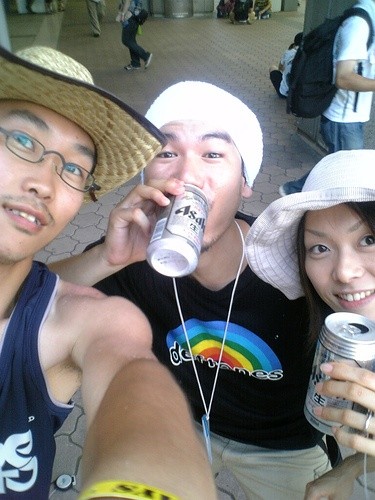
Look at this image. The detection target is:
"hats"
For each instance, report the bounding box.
[244,149,375,301]
[144,81,264,187]
[1,45,168,206]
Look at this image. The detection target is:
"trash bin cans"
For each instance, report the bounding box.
[165,0,193,17]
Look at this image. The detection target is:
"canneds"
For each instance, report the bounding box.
[146,184,208,278]
[304,312,375,437]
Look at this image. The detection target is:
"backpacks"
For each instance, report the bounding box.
[286,7,374,118]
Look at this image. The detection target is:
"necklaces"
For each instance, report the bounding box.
[173,218,245,463]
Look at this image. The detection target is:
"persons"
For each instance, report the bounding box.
[115,1,152,71]
[243,149,375,500]
[86,1,105,37]
[44,81,343,499]
[0,45,217,500]
[24,0,52,15]
[279,0,375,196]
[270,32,303,99]
[216,0,272,25]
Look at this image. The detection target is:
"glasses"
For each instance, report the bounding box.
[0,126,101,192]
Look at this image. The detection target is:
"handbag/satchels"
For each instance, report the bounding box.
[137,10,148,25]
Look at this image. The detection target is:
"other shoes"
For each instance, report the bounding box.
[144,53,153,68]
[124,63,141,70]
[262,15,270,19]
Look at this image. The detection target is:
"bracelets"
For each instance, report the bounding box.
[76,480,182,500]
